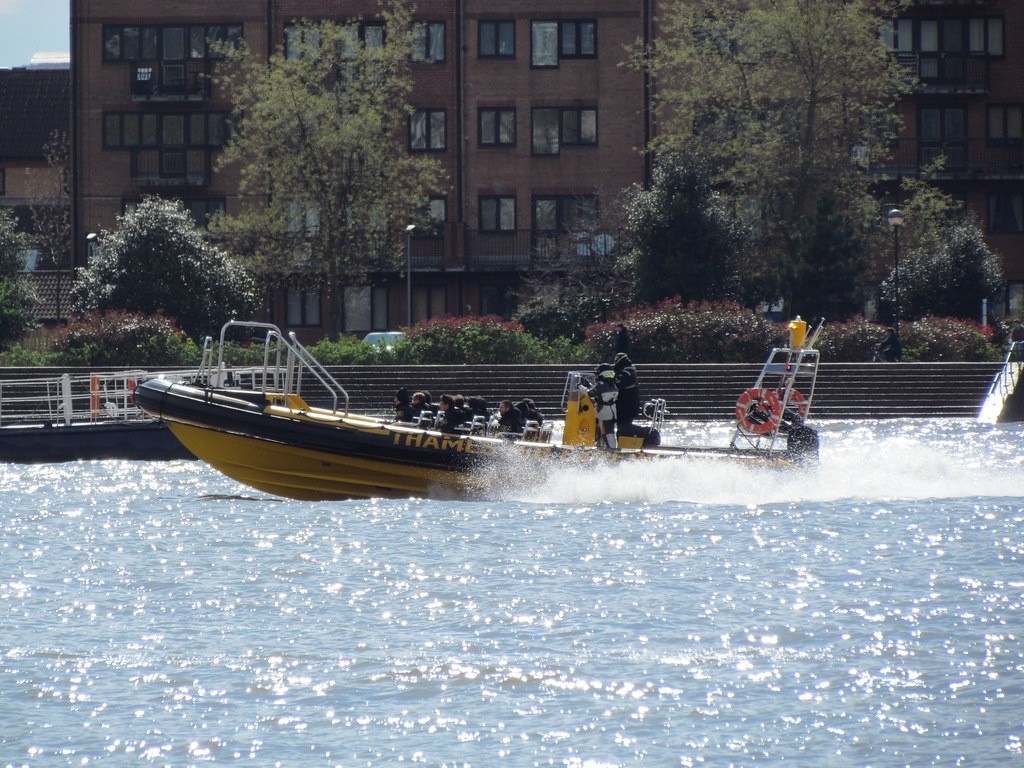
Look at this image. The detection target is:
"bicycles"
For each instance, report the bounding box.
[867,343,918,362]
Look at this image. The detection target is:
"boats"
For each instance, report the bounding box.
[130,313,828,502]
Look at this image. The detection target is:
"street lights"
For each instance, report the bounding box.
[886,209,907,341]
[405,224,416,331]
[86,232,100,348]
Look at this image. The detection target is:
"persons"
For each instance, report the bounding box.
[1011,318,1024,361]
[879,326,902,362]
[422,389,440,426]
[516,398,542,436]
[614,324,632,354]
[453,394,473,421]
[395,387,420,424]
[498,401,525,440]
[410,392,432,427]
[582,370,619,451]
[469,397,490,422]
[439,394,465,434]
[613,351,662,446]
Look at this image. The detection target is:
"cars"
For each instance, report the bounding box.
[358,330,412,357]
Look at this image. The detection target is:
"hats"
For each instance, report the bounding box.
[594,363,610,374]
[396,388,408,403]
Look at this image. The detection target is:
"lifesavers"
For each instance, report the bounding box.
[735,387,781,435]
[771,386,807,434]
